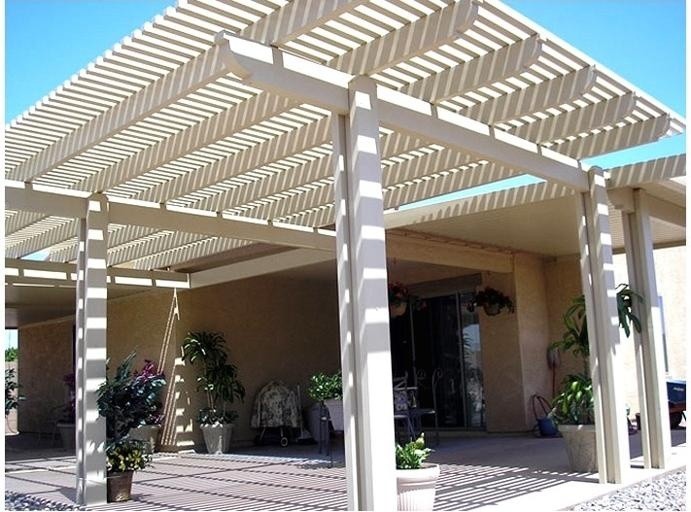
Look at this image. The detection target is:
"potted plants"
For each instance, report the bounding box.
[549,374,597,473]
[106,438,152,503]
[394,432,439,512]
[309,368,344,433]
[181,328,246,454]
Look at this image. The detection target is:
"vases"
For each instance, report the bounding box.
[127,422,161,456]
[56,421,75,451]
[390,301,407,315]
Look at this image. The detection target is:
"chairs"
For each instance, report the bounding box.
[393,389,440,449]
[316,402,330,456]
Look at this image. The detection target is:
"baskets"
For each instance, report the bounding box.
[390,302,407,318]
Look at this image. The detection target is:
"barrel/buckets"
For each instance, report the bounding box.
[536,417,556,436]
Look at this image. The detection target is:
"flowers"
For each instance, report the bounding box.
[55,374,78,425]
[388,280,426,310]
[464,287,514,315]
[130,357,165,425]
